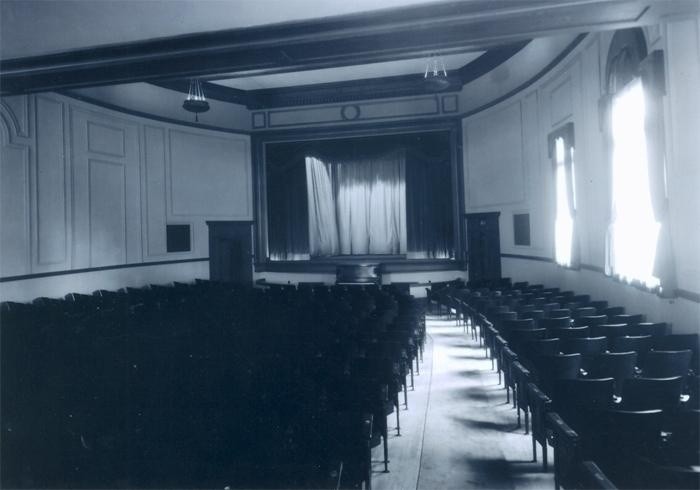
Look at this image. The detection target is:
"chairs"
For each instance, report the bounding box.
[2,277,427,490]
[421,270,700,490]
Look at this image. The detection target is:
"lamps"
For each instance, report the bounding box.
[422,58,450,93]
[184,78,210,123]
